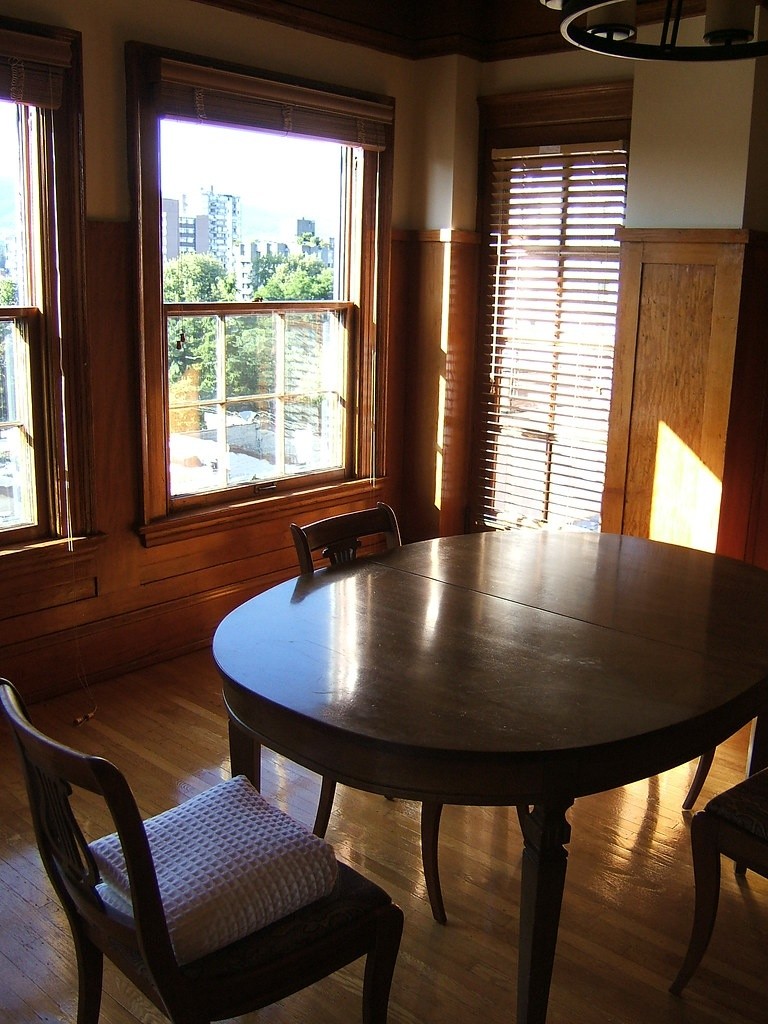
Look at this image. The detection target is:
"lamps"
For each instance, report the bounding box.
[540,0,768,61]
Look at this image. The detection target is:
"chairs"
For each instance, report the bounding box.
[667,767,768,996]
[293,502,448,926]
[0,676,404,1024]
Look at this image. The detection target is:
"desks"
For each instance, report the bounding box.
[210,529,768,1024]
